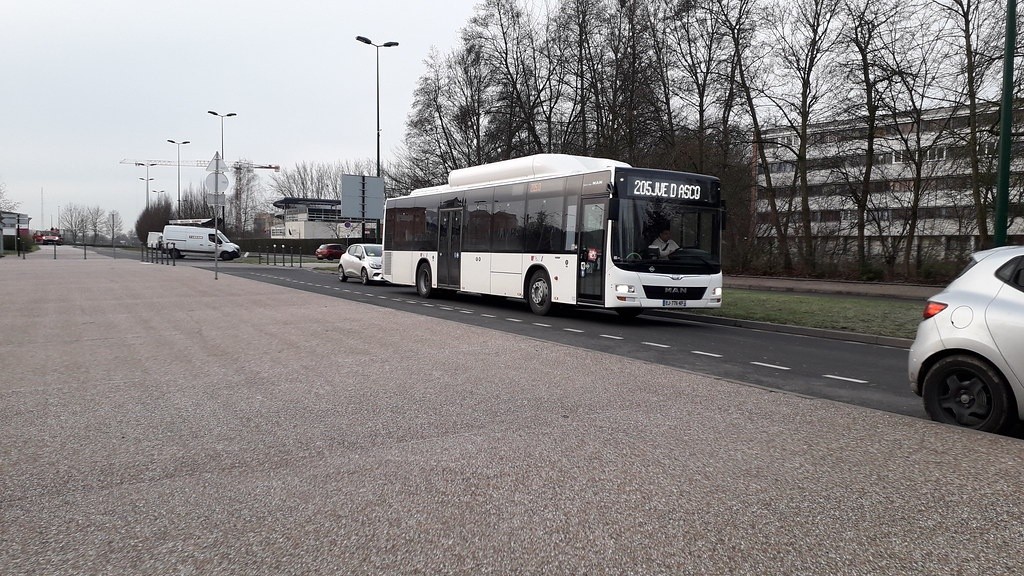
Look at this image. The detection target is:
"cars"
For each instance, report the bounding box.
[33,231,61,245]
[907,246,1024,435]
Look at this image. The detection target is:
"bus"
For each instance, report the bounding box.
[381,153,728,322]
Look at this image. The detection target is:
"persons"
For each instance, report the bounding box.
[651,228,681,260]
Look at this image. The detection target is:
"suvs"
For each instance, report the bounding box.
[337,244,390,286]
[316,244,346,261]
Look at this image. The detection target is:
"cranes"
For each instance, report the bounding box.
[120,156,281,234]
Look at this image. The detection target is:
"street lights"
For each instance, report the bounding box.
[208,111,237,162]
[356,34,399,177]
[167,140,190,221]
[152,190,164,207]
[140,177,154,210]
[138,162,156,212]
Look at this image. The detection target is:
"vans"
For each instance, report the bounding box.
[147,232,163,249]
[161,218,242,261]
